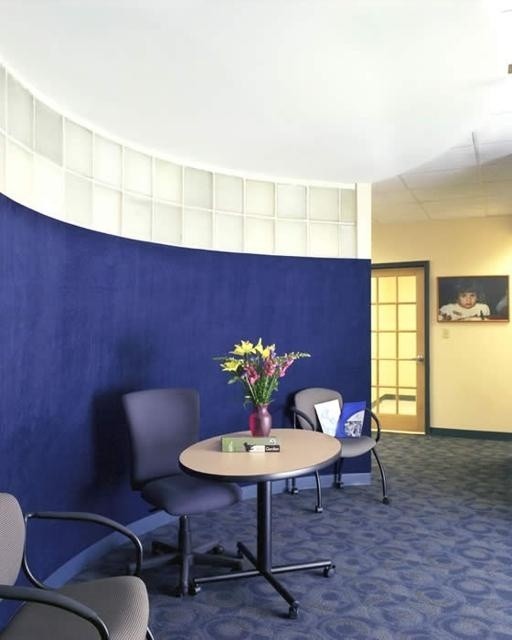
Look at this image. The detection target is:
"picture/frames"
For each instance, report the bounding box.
[436,275,510,323]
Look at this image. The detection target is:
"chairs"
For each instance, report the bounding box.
[122,389,244,597]
[0,492,153,639]
[292,387,390,513]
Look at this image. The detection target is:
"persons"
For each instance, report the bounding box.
[439,285,491,321]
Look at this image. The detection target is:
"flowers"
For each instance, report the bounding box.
[212,337,312,435]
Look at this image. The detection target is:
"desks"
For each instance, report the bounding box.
[179,427,342,620]
